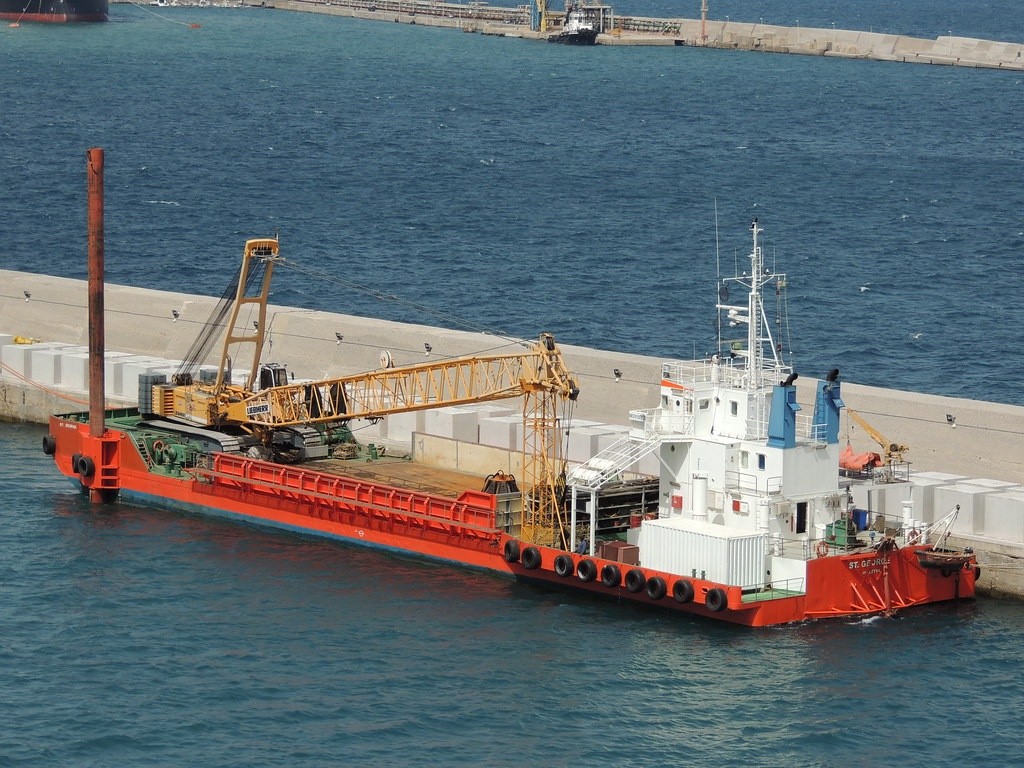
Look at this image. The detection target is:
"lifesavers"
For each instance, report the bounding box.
[672,579,695,603]
[153,440,164,453]
[601,564,621,588]
[77,456,93,476]
[410,20,416,25]
[941,567,953,577]
[9,20,20,31]
[645,577,667,600]
[395,18,398,22]
[624,569,645,594]
[553,554,574,578]
[42,435,56,454]
[505,540,520,563]
[812,540,832,559]
[71,454,83,474]
[409,12,415,17]
[975,564,982,582]
[521,547,541,570]
[366,5,376,13]
[186,20,202,31]
[704,587,728,613]
[905,532,921,544]
[576,559,597,582]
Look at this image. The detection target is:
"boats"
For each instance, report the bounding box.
[546,0,601,45]
[40,146,981,627]
[0,0,107,22]
